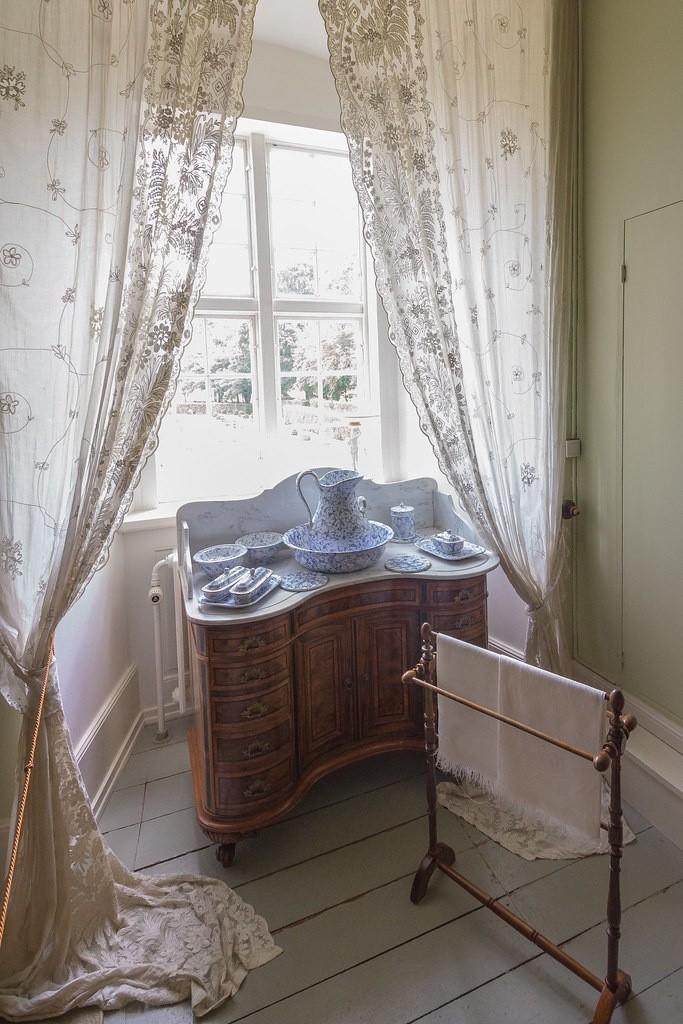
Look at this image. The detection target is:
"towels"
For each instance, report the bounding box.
[429,631,610,848]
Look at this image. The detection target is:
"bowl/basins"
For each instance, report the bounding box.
[283,520,394,573]
[235,532,283,566]
[193,544,248,580]
[432,530,465,555]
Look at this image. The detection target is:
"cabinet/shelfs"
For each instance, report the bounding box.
[174,466,502,869]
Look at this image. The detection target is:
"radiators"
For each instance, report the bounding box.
[148,552,193,746]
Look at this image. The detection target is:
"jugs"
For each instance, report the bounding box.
[296,470,373,550]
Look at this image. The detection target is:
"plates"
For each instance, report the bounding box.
[415,539,486,560]
[198,575,282,608]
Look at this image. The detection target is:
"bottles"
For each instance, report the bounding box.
[391,503,415,540]
